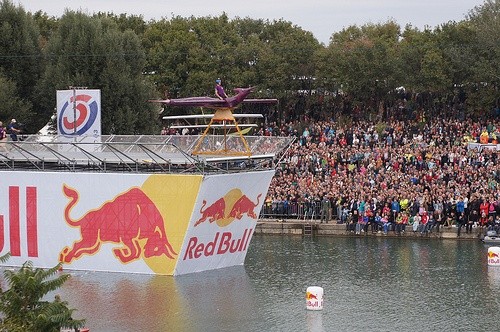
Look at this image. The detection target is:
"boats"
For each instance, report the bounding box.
[0,128,299,277]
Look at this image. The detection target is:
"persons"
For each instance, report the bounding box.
[7,119,21,141]
[215,78,229,100]
[252,118,500,239]
[161,121,251,170]
[0,120,7,141]
